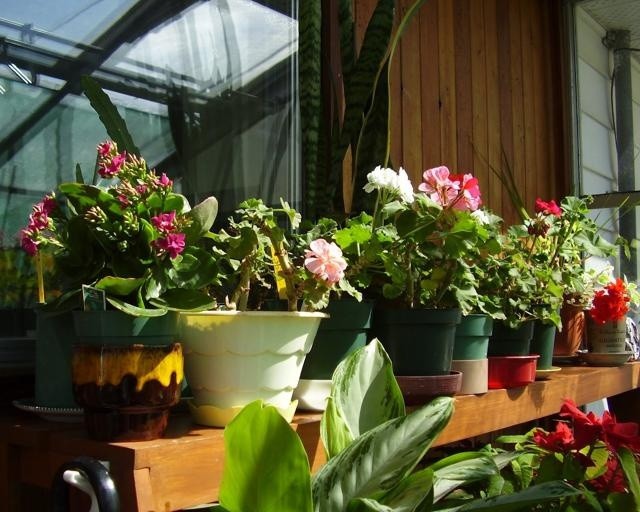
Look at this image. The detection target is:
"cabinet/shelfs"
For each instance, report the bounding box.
[104,357,640,512]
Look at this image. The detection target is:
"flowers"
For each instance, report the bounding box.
[589,277,632,323]
[528,197,561,236]
[21,142,193,269]
[550,404,638,490]
[304,241,348,284]
[362,160,483,219]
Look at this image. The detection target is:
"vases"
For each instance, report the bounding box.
[533,316,559,378]
[458,315,494,396]
[304,286,373,414]
[591,319,634,365]
[59,297,186,440]
[370,303,462,396]
[486,314,541,388]
[553,290,586,366]
[185,283,318,425]
